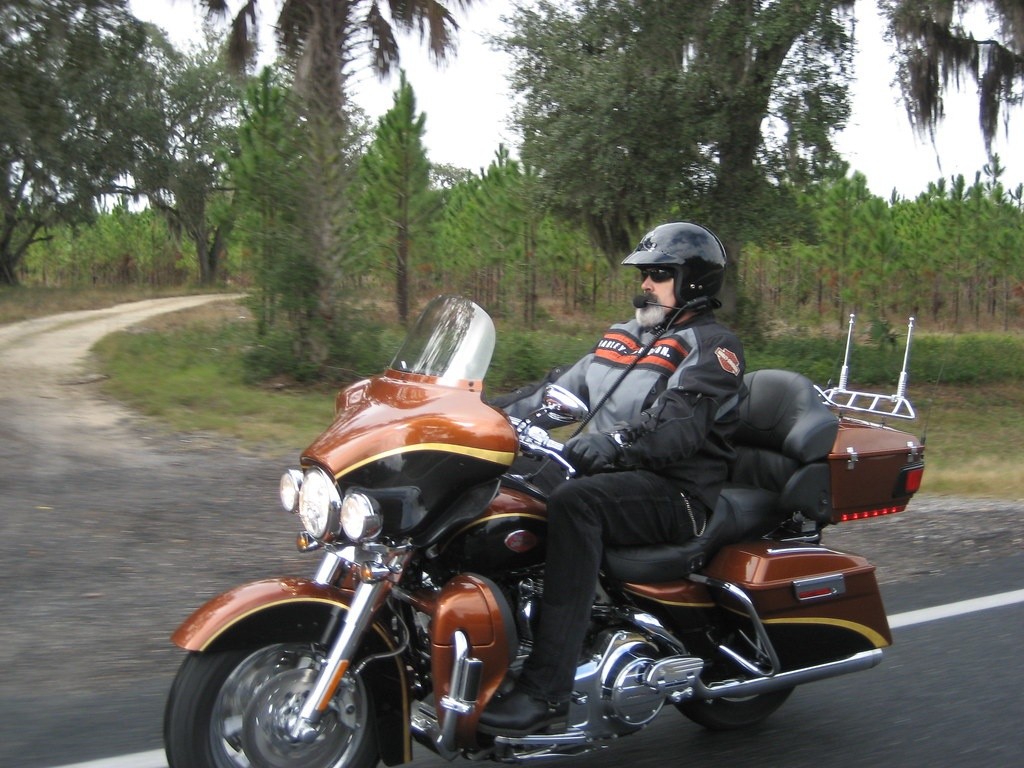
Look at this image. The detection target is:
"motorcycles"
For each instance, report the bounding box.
[163,295,946,768]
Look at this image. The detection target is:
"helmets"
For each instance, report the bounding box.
[621,222,727,311]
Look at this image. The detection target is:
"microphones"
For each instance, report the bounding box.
[633,295,706,312]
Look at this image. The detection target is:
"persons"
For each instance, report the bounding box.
[475,221,750,738]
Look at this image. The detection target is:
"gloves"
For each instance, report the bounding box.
[561,420,637,477]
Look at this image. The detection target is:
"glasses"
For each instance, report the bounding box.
[640,266,675,283]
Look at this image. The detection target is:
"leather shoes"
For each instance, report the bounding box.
[477,689,570,738]
